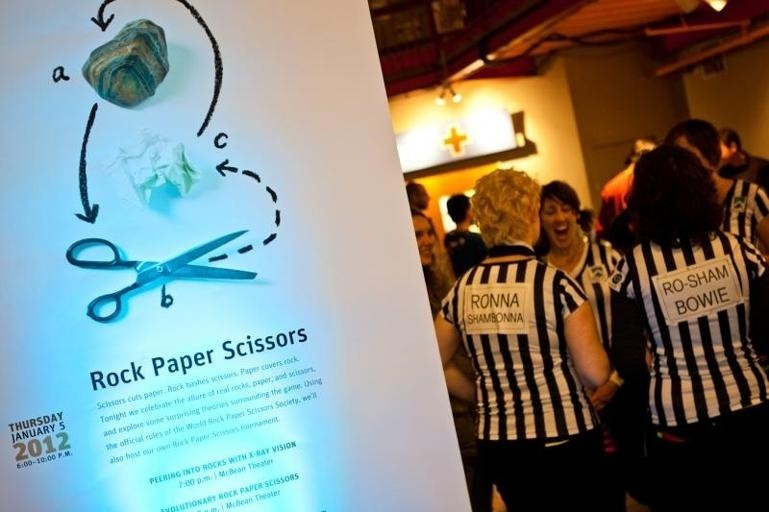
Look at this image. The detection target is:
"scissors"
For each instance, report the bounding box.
[65,230,258,322]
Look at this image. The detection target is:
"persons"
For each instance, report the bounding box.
[405,117,768,511]
